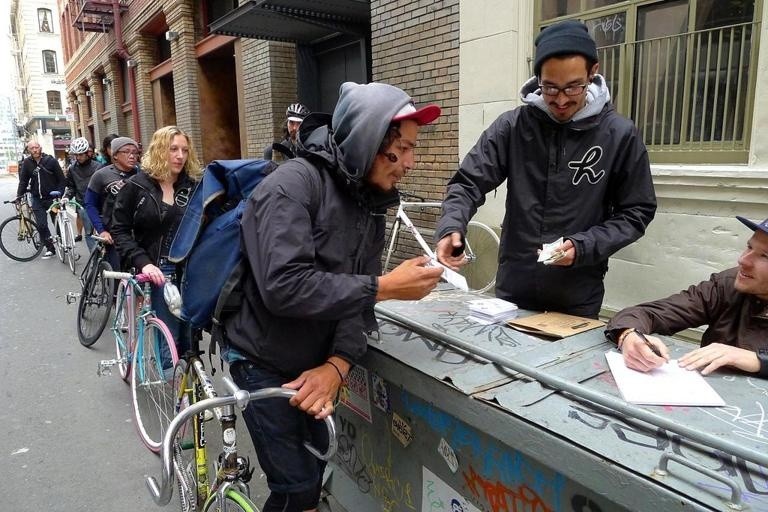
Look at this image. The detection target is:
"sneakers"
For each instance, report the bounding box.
[42,235,82,259]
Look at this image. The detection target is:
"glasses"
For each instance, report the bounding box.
[537,75,593,96]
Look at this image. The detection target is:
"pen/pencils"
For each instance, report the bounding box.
[634,328,668,365]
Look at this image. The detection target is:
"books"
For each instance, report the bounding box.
[604,350,726,407]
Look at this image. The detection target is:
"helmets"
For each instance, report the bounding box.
[286,103,311,119]
[68,136,89,155]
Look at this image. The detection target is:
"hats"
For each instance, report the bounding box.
[534,22,598,77]
[111,137,140,162]
[736,215,768,234]
[393,102,441,126]
[287,116,303,122]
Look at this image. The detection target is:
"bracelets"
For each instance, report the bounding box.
[612,327,641,351]
[323,359,344,383]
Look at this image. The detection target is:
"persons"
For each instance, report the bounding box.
[15,125,218,425]
[605,209,768,380]
[434,17,656,322]
[261,101,313,164]
[210,78,444,511]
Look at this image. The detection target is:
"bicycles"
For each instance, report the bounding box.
[0,188,45,263]
[145,323,338,511]
[102,267,190,453]
[45,191,85,275]
[383,185,502,293]
[65,233,115,348]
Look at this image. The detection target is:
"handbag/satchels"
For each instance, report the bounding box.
[169,159,278,332]
